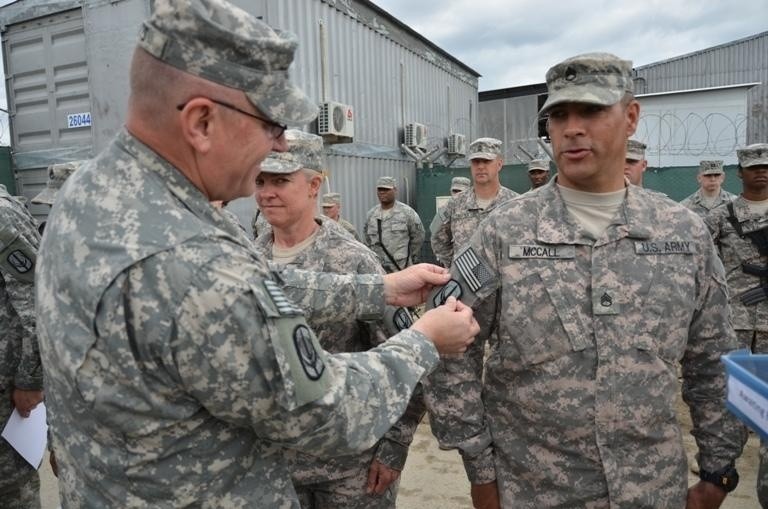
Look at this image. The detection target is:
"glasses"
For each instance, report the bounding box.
[176,100,286,139]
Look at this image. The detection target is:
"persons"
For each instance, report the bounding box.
[622,139,768,354]
[252,131,423,509]
[424,52,750,508]
[34,0,481,508]
[429,137,550,266]
[1,159,90,509]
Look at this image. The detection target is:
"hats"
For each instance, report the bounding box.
[31,160,89,203]
[527,159,549,172]
[624,140,646,161]
[699,161,722,176]
[376,177,397,191]
[450,177,470,191]
[735,143,767,168]
[538,52,632,115]
[259,129,323,173]
[137,0,319,127]
[319,192,340,207]
[467,138,501,161]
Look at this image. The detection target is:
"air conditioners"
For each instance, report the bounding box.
[403,121,428,150]
[317,99,354,137]
[447,133,467,156]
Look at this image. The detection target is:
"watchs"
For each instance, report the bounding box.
[700,468,739,491]
